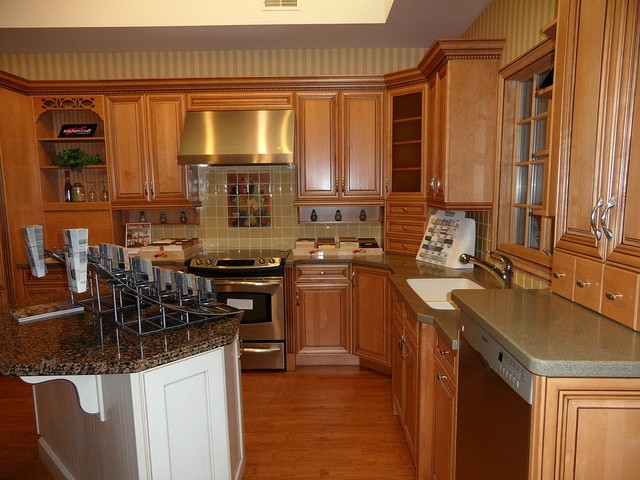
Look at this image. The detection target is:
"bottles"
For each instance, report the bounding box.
[65,170,72,202]
[335,209,343,221]
[310,209,318,222]
[139,211,146,225]
[360,209,366,221]
[159,212,167,224]
[180,210,187,224]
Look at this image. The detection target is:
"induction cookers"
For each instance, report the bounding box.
[189,249,294,274]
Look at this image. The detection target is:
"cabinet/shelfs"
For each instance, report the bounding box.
[18,258,110,301]
[0,69,114,212]
[346,264,392,375]
[284,263,352,368]
[497,37,556,270]
[550,0,640,334]
[294,74,383,206]
[430,326,458,480]
[105,79,189,210]
[388,274,432,480]
[454,309,639,480]
[383,67,429,257]
[417,41,505,210]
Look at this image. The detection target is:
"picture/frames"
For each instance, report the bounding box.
[125,221,152,249]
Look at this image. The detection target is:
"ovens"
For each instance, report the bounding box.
[190,266,284,348]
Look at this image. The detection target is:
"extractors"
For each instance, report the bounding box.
[174,106,299,168]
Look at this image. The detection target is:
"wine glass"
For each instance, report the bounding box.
[100,181,109,200]
[87,182,98,200]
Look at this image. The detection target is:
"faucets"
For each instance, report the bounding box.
[460,251,514,289]
[405,276,485,310]
